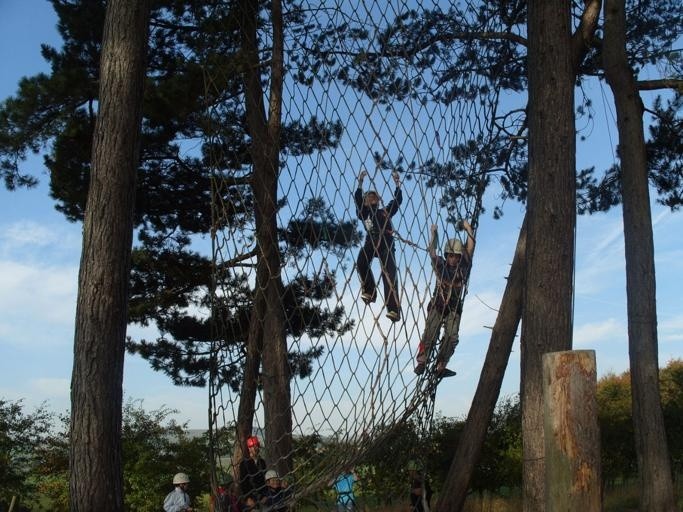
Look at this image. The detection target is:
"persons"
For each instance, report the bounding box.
[237,435,268,510]
[206,468,236,512]
[326,460,362,511]
[413,218,475,380]
[242,495,258,511]
[403,455,433,511]
[351,170,405,324]
[256,466,291,512]
[161,472,194,511]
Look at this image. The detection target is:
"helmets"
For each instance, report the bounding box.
[444,238,463,256]
[172,472,191,485]
[364,190,380,200]
[265,469,280,481]
[246,436,259,447]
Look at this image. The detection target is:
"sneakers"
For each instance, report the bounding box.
[414,363,425,375]
[434,367,456,377]
[360,291,375,302]
[385,309,401,322]
